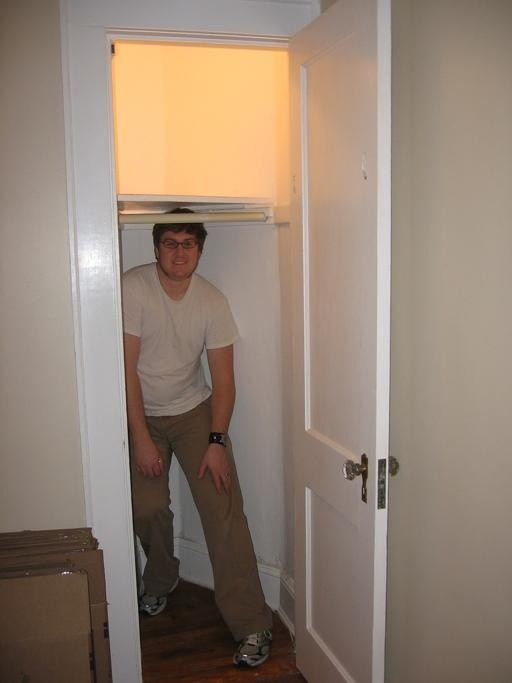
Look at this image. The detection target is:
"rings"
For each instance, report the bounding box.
[225,474,229,481]
[157,458,162,464]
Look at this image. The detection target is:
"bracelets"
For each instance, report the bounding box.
[208,432,228,447]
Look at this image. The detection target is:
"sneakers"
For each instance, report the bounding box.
[139,573,180,617]
[232,629,273,667]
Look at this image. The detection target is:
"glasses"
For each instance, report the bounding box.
[156,237,203,249]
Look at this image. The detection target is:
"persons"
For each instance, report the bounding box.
[123,209,273,667]
